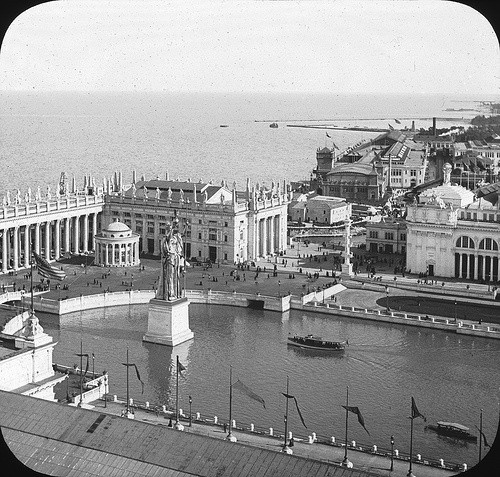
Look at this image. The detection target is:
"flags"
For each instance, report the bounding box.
[31,250,68,281]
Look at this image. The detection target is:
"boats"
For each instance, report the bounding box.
[288,334,345,350]
[426,421,477,439]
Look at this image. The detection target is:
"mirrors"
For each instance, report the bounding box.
[0,0,500,477]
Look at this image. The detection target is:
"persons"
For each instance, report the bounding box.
[155,209,189,300]
